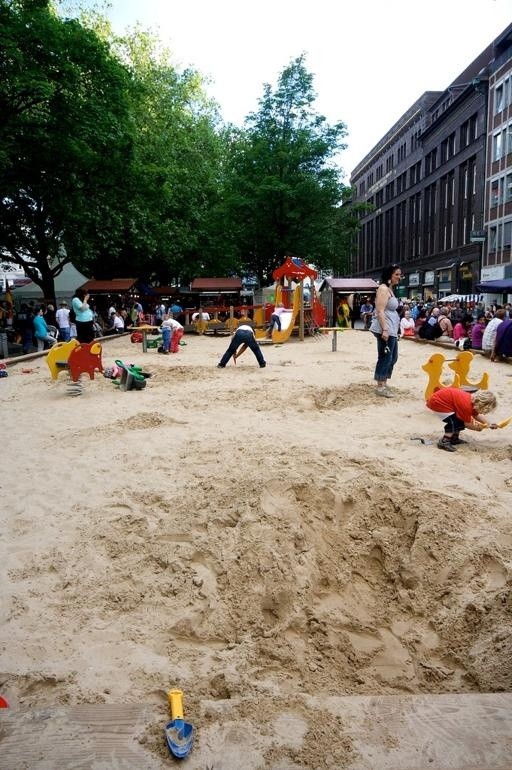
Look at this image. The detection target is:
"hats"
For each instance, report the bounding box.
[59,301,67,305]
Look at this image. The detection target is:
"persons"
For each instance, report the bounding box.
[191,307,210,335]
[0,287,184,360]
[217,324,266,368]
[369,263,400,398]
[267,302,287,337]
[398,295,512,362]
[360,300,373,330]
[426,388,497,452]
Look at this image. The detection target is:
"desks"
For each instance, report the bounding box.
[318,327,352,352]
[126,326,160,353]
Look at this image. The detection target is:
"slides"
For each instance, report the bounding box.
[255,308,299,343]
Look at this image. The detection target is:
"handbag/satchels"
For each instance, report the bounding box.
[418,322,431,338]
[404,327,415,336]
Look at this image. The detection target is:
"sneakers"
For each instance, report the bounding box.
[437,439,456,452]
[376,387,392,398]
[451,438,467,444]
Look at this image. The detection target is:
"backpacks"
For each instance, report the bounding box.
[431,317,447,337]
